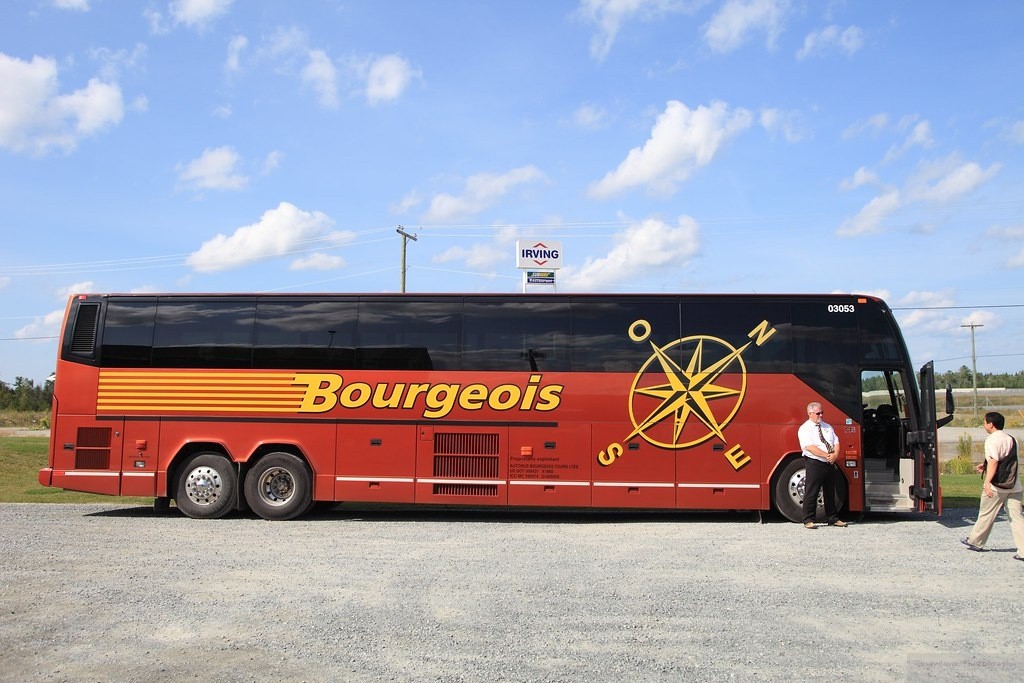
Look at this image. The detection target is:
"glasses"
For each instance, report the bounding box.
[814,411,824,415]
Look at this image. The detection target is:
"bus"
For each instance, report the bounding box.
[39,290,955,519]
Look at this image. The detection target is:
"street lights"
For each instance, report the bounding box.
[959,323,985,416]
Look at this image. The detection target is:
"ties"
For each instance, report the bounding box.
[816,425,839,455]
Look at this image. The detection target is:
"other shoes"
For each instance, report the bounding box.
[833,520,849,526]
[1014,554,1024,561]
[806,521,819,528]
[960,537,983,551]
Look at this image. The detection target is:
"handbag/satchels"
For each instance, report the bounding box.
[980,433,1019,489]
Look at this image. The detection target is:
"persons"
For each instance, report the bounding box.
[960,412,1024,561]
[798,402,848,529]
